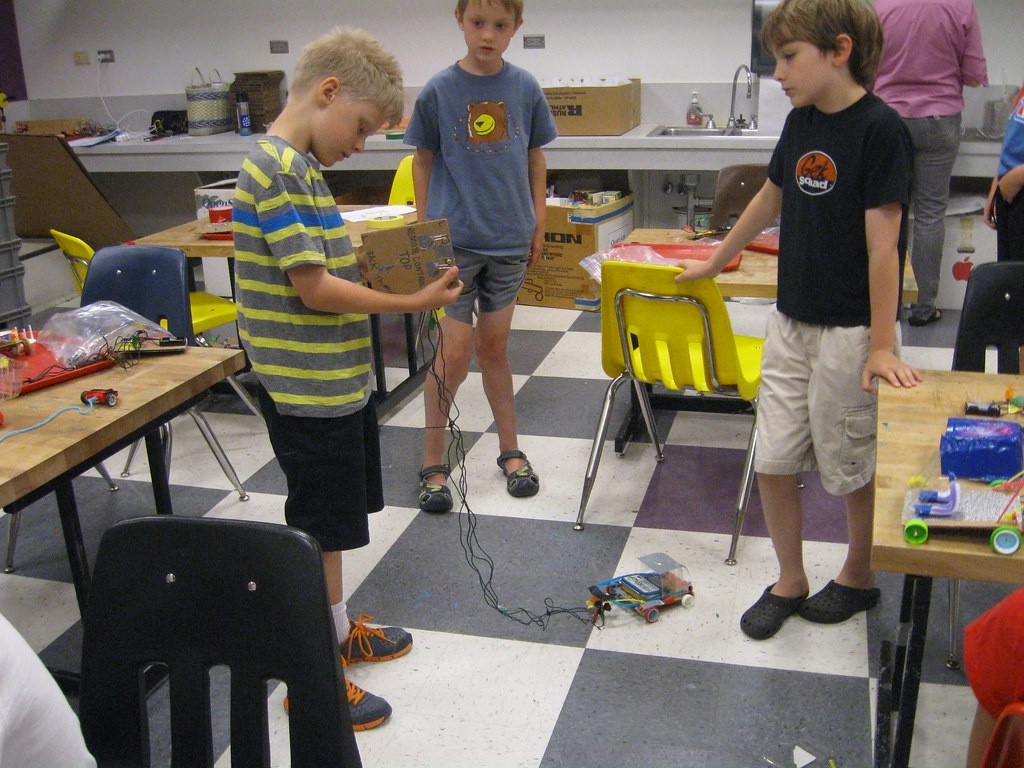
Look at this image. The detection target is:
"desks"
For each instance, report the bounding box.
[123,205,438,414]
[868,369,1024,768]
[0,329,253,629]
[608,228,920,452]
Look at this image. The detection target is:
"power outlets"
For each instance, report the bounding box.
[97,49,115,63]
[523,35,545,49]
[270,41,290,54]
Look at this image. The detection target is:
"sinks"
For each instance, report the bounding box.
[727,128,758,136]
[646,125,727,136]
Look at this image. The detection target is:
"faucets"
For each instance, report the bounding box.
[726,64,753,128]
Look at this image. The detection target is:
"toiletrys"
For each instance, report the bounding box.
[687,91,703,125]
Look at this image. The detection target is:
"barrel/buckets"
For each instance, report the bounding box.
[675,207,712,230]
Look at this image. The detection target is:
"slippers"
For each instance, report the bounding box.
[799,580,881,623]
[741,583,809,641]
[908,308,942,326]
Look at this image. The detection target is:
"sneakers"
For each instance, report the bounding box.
[339,614,413,664]
[284,679,391,731]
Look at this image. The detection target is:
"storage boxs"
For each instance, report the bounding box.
[539,78,640,135]
[515,194,632,311]
[902,196,1000,313]
[0,142,34,332]
[193,178,239,237]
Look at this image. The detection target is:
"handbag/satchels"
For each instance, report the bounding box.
[185,68,235,136]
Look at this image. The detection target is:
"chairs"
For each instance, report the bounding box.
[78,516,363,768]
[574,258,765,566]
[946,261,1023,671]
[385,157,446,356]
[68,244,248,506]
[43,229,271,430]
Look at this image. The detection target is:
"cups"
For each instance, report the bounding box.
[209,207,232,223]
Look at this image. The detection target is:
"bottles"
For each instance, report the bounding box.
[235,92,252,135]
[572,189,584,206]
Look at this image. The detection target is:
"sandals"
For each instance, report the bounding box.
[419,465,453,511]
[496,450,539,497]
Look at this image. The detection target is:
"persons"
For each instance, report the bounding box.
[674,0,923,640]
[0,614,99,768]
[983,86,1024,375]
[403,0,559,515]
[233,28,465,731]
[964,588,1024,768]
[874,1,989,329]
